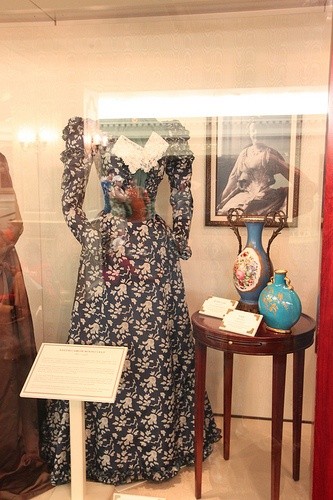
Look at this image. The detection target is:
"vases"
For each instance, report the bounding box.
[226,208,286,311]
[258,269,302,335]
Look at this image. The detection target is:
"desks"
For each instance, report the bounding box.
[189,302,316,500]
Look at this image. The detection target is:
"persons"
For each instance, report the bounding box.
[218,119,288,217]
[0,110,222,500]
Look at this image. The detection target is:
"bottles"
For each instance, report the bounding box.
[233,218,272,304]
[257,270,302,333]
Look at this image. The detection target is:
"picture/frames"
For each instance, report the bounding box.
[204,113,303,230]
